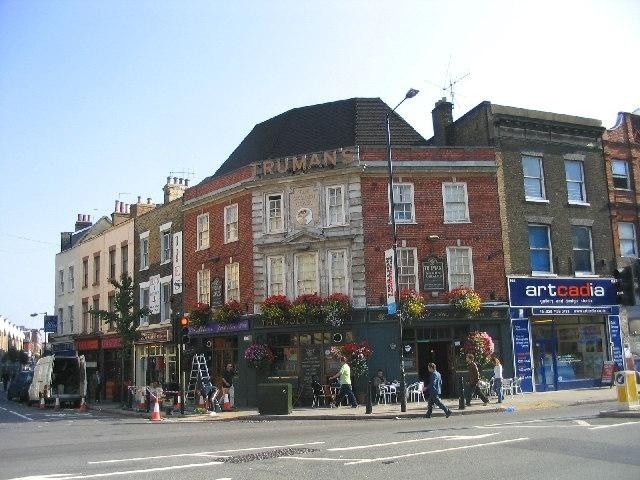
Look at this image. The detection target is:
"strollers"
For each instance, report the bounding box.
[311,380,336,409]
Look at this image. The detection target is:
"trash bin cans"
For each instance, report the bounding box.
[257,383,292,414]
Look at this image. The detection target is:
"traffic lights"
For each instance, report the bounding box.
[613,266,635,306]
[178,316,189,344]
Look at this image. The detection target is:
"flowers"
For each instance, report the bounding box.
[245,344,275,361]
[329,338,374,378]
[261,292,353,327]
[460,329,494,365]
[446,285,482,318]
[397,288,429,320]
[187,299,243,326]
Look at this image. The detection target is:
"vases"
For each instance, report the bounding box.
[248,359,270,369]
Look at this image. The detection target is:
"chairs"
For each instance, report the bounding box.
[309,381,353,408]
[474,377,524,399]
[369,379,426,404]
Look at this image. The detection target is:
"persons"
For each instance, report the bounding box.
[422,363,452,418]
[373,369,390,404]
[330,370,347,406]
[221,362,234,408]
[91,369,102,405]
[463,354,490,406]
[328,356,358,407]
[310,375,332,407]
[490,357,504,403]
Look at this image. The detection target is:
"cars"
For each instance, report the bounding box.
[7,370,34,403]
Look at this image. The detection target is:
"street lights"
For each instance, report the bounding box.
[385,88,420,411]
[30,312,47,355]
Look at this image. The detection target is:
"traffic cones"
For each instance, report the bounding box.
[198,396,205,408]
[223,393,232,412]
[137,395,147,413]
[80,398,87,412]
[54,397,61,412]
[176,396,183,411]
[150,396,162,422]
[40,396,46,410]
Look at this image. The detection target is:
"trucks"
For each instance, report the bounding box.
[27,355,87,407]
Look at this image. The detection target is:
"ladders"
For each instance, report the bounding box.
[185,354,221,412]
